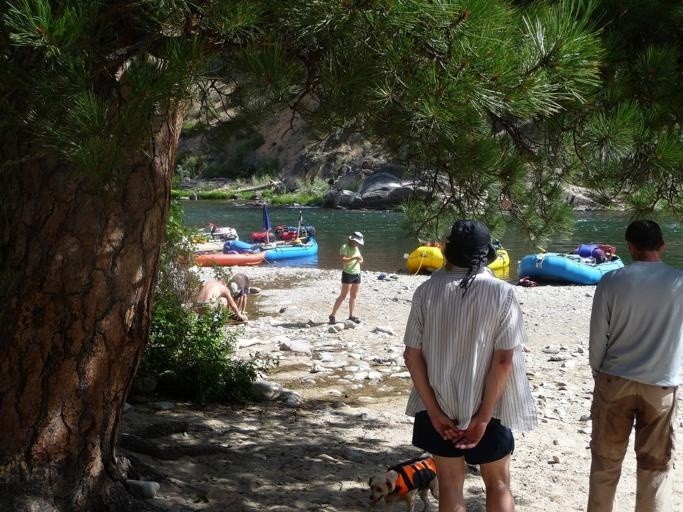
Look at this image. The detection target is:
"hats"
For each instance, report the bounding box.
[348,232,365,246]
[442,218,497,271]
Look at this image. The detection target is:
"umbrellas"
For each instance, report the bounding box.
[261,201,272,243]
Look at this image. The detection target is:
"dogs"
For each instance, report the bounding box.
[367,452,439,512]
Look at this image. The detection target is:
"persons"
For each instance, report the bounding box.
[403,222,539,512]
[194,275,245,321]
[227,274,249,315]
[587,219,682,512]
[330,231,365,324]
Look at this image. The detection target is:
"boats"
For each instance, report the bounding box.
[516,244,624,286]
[174,223,317,268]
[403,231,510,274]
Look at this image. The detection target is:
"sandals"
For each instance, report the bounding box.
[349,316,361,324]
[328,315,335,324]
